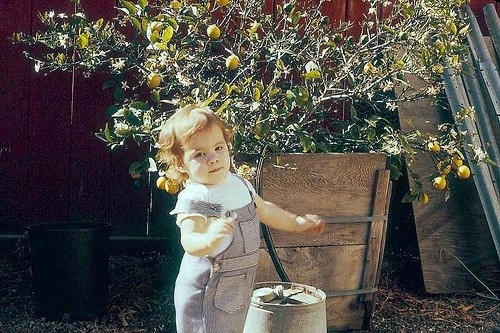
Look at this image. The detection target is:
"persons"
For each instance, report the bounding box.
[156,104,326,333]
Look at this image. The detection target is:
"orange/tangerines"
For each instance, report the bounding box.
[145,0,471,204]
